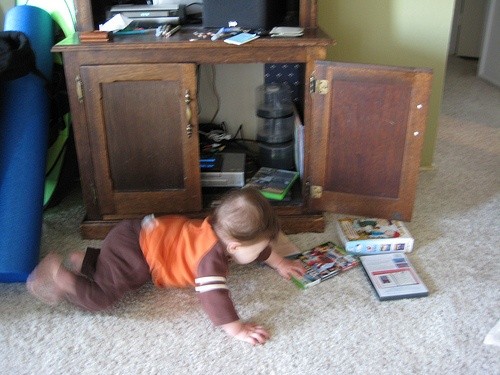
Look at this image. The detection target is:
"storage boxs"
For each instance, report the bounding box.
[335,217,414,256]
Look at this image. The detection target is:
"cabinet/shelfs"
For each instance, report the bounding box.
[50,25,433,241]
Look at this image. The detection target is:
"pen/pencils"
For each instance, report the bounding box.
[211,26,226,41]
[155,24,181,38]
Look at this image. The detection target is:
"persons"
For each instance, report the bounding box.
[27,188,306,346]
